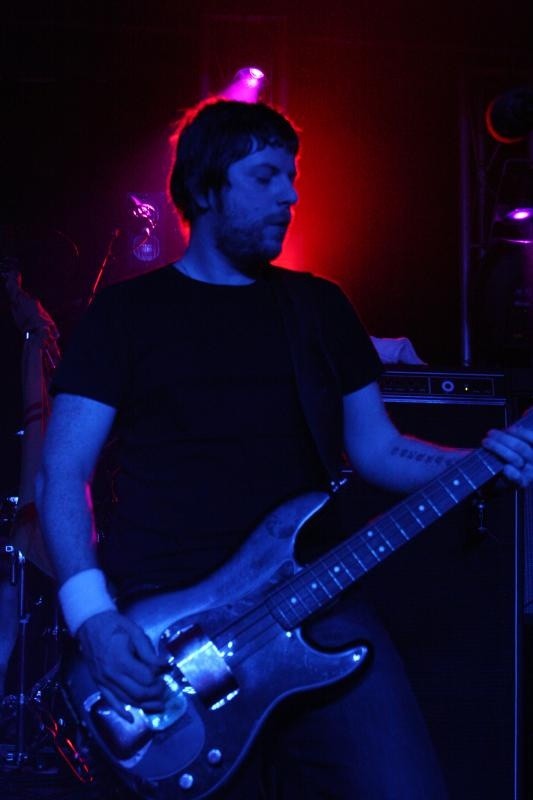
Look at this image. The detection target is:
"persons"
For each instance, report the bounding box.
[0,260,59,699]
[35,101,533,800]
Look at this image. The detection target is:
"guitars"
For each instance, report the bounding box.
[34,414,532,768]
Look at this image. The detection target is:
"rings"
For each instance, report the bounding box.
[520,460,527,470]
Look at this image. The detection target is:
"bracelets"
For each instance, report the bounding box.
[57,569,117,636]
